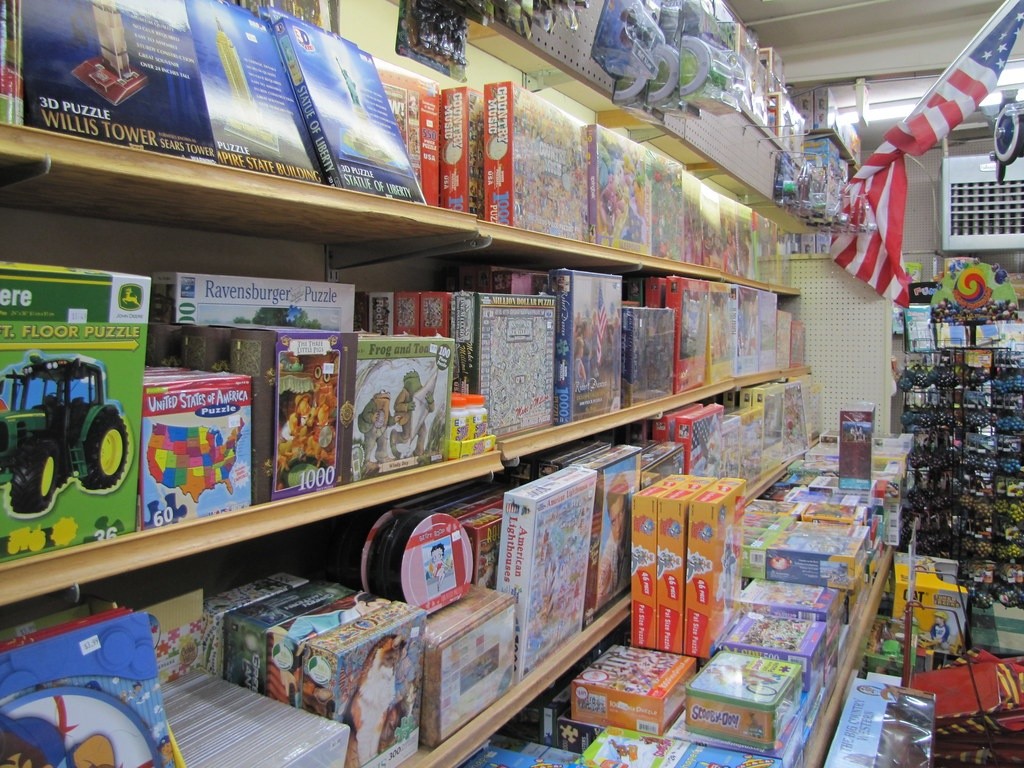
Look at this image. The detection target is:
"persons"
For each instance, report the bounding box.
[597,473,631,608]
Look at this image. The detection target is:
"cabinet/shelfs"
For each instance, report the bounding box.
[1,120,822,768]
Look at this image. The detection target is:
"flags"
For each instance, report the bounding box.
[829,0,1024,309]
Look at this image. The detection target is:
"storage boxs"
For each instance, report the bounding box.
[137,1,970,768]
[18,1,218,164]
[1,260,152,564]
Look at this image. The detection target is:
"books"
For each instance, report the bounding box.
[0,0,426,204]
